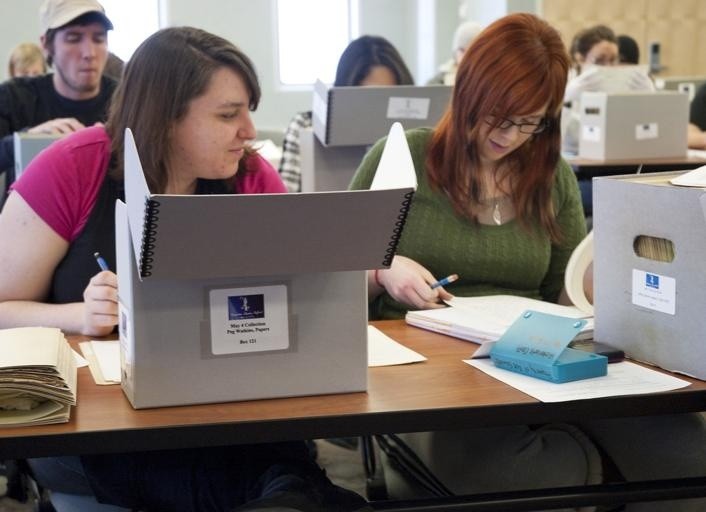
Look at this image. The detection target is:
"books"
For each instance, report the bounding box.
[0,327,77,427]
[405,228,593,344]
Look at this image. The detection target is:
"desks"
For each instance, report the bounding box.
[0,319,706,511]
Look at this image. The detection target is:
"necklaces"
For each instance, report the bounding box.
[480,160,518,225]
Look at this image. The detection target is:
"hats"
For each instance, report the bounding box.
[42,0,114,33]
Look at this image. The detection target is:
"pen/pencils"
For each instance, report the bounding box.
[93,251,109,271]
[431,274,459,289]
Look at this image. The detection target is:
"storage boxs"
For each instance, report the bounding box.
[656,79,706,103]
[592,170,706,381]
[300,83,455,193]
[102,125,418,409]
[580,92,691,163]
[14,131,67,179]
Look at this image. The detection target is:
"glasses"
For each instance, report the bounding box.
[484,114,546,134]
[588,54,620,65]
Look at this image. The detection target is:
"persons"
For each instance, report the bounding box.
[0,0,121,210]
[280,36,413,192]
[566,24,705,149]
[349,14,593,511]
[0,26,369,512]
[425,21,482,84]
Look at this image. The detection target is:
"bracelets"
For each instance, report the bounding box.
[375,267,385,289]
